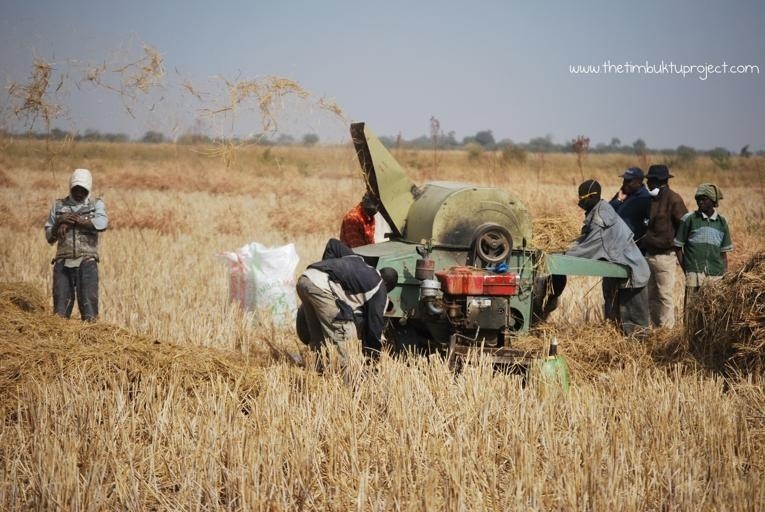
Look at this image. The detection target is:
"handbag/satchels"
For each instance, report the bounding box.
[334,299,355,322]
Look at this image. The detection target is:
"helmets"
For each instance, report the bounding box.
[618,167,644,180]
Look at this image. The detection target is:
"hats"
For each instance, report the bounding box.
[362,191,376,209]
[644,164,674,181]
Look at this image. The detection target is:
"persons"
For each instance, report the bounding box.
[340,191,379,248]
[637,165,688,330]
[671,182,735,328]
[549,178,649,325]
[296,239,399,385]
[608,166,651,259]
[43,168,109,323]
